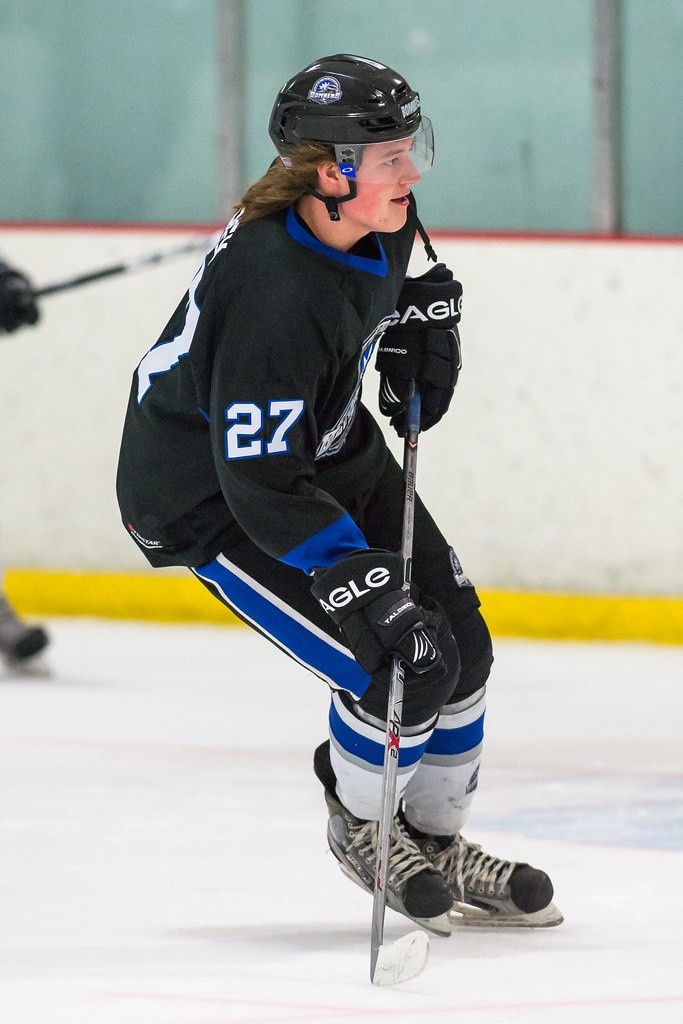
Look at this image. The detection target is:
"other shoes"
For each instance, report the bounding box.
[0,594,48,663]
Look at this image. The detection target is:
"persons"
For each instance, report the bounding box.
[117,52,563,938]
[0,262,49,660]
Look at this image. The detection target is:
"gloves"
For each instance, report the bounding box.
[310,548,448,695]
[0,262,39,332]
[375,263,463,438]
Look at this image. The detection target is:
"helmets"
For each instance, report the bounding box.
[268,54,422,172]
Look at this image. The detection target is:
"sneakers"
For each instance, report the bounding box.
[405,812,564,927]
[324,788,454,937]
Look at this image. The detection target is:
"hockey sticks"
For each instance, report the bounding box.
[363,355,437,988]
